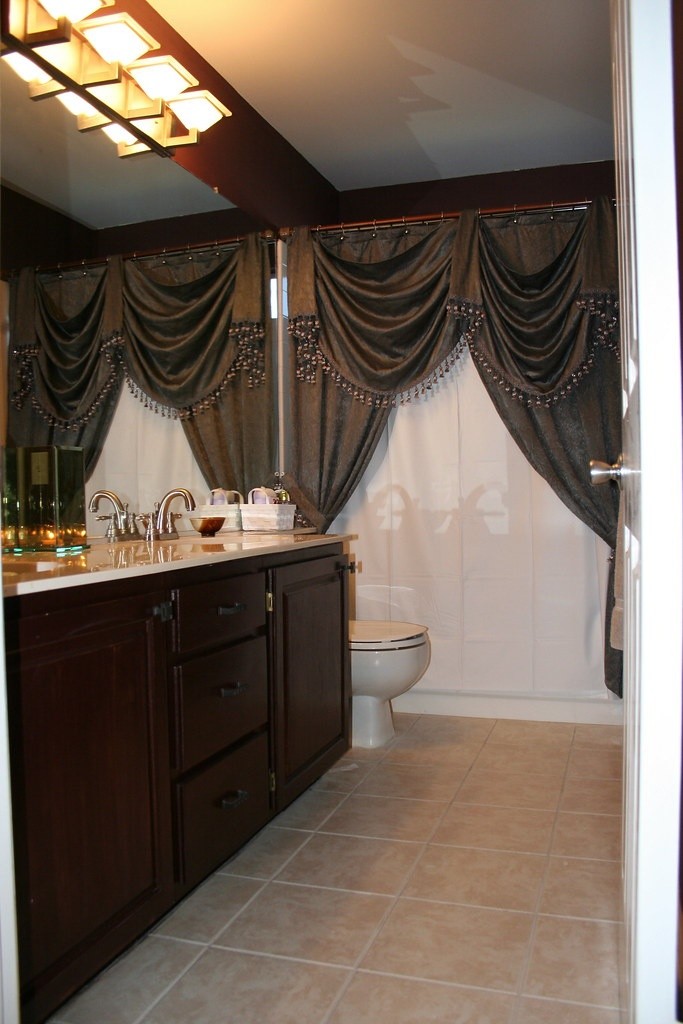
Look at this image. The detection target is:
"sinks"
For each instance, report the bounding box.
[2,562,68,578]
[166,537,293,545]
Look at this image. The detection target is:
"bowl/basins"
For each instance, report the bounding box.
[189,517,225,538]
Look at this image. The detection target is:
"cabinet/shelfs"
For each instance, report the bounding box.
[3,540,355,1024]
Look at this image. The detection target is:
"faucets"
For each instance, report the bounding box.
[87,491,129,535]
[157,488,196,533]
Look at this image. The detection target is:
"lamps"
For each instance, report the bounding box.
[0,1,231,157]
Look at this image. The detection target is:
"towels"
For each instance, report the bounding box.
[610,489,624,652]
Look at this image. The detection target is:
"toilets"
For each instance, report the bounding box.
[348,618,431,749]
